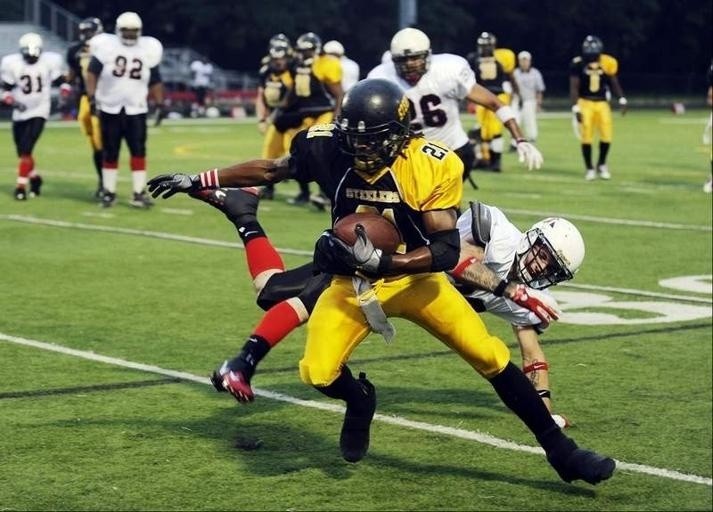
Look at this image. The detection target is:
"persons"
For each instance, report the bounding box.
[570,36,628,180]
[703,65,713,193]
[1,33,65,200]
[86,12,165,208]
[66,16,104,197]
[510,51,546,152]
[187,187,584,427]
[147,78,616,486]
[366,28,543,219]
[190,32,359,210]
[467,32,523,171]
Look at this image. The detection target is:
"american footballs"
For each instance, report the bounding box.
[333,212,401,255]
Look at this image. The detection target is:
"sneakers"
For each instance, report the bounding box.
[340,373,375,462]
[548,437,615,483]
[15,176,41,200]
[586,164,610,180]
[189,187,258,217]
[211,360,253,403]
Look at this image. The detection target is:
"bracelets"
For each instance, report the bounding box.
[572,105,579,113]
[618,97,627,105]
[493,279,510,297]
[537,390,550,397]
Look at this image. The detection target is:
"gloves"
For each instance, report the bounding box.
[315,225,393,281]
[511,284,560,324]
[147,174,201,198]
[517,139,543,171]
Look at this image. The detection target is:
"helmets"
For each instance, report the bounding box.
[269,33,344,73]
[515,217,585,290]
[20,33,41,64]
[78,12,143,46]
[391,28,430,76]
[580,36,602,62]
[477,32,496,54]
[331,79,409,176]
